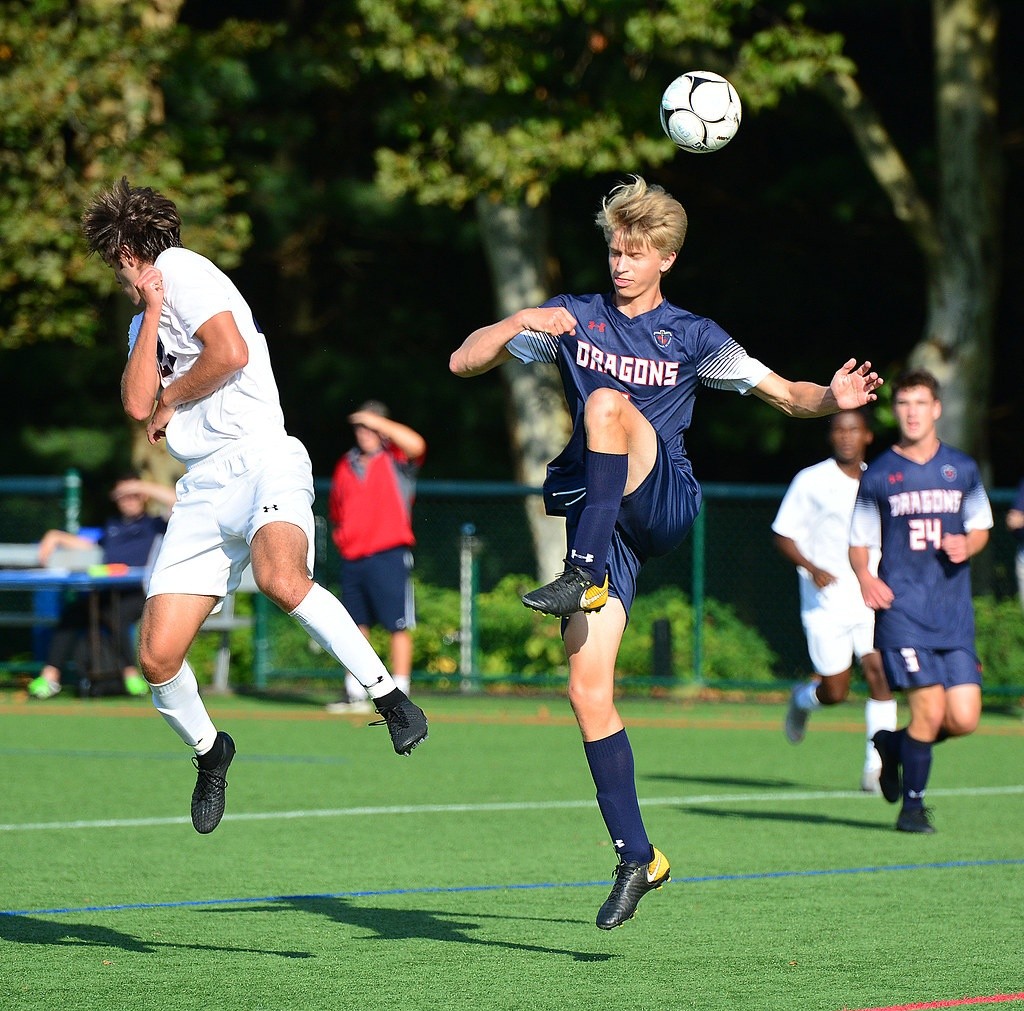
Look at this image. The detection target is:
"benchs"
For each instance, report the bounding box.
[0,534,260,692]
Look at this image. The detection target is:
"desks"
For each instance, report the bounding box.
[0,567,149,697]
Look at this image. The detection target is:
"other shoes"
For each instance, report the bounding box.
[873,730,902,804]
[123,674,148,696]
[785,682,810,746]
[897,806,936,833]
[28,675,62,699]
[862,770,881,793]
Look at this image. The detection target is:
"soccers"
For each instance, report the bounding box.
[657,69,744,156]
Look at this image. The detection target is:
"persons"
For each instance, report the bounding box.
[27,470,184,702]
[325,402,425,715]
[83,177,427,835]
[848,366,994,834]
[448,176,882,930]
[1006,493,1024,600]
[771,410,897,795]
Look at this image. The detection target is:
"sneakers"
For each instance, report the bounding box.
[368,698,429,757]
[595,843,672,931]
[521,559,608,618]
[190,731,236,834]
